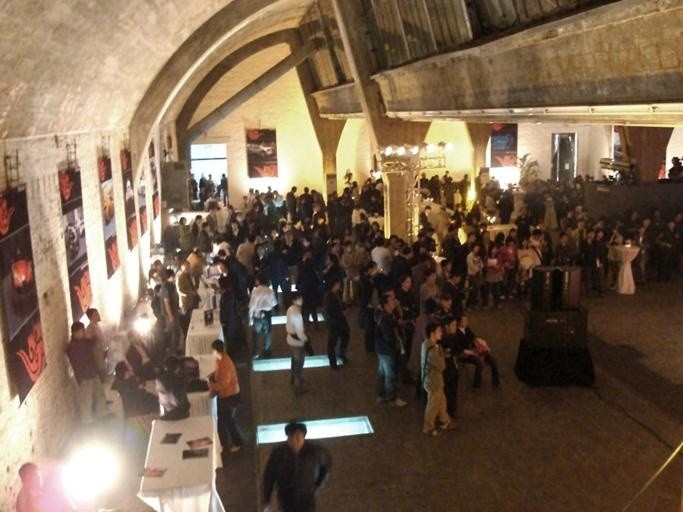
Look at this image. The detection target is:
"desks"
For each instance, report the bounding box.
[611,243,640,295]
[135,261,226,511]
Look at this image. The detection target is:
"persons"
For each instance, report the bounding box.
[62,156,682,452]
[260,424,332,511]
[14,462,70,511]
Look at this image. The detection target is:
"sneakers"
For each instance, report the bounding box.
[423,420,456,437]
[376,395,408,407]
[230,446,240,451]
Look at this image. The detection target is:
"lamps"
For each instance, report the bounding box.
[373,139,454,160]
